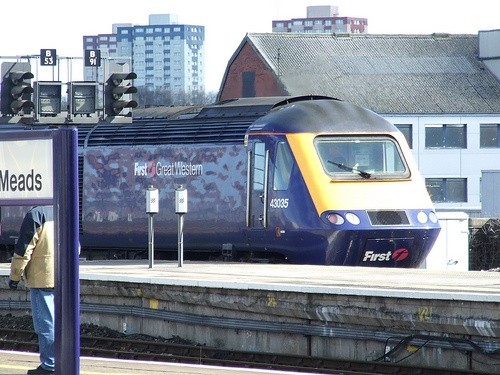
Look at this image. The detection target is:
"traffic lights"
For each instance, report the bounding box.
[1,62,35,114]
[38,81,63,114]
[105,62,138,118]
[71,81,99,114]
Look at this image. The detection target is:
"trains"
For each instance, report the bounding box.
[0,93,442,269]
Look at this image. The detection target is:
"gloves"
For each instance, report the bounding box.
[8,280,19,289]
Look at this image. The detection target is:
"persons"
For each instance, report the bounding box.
[8,203,82,375]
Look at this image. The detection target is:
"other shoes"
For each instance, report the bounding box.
[27,364,55,375]
